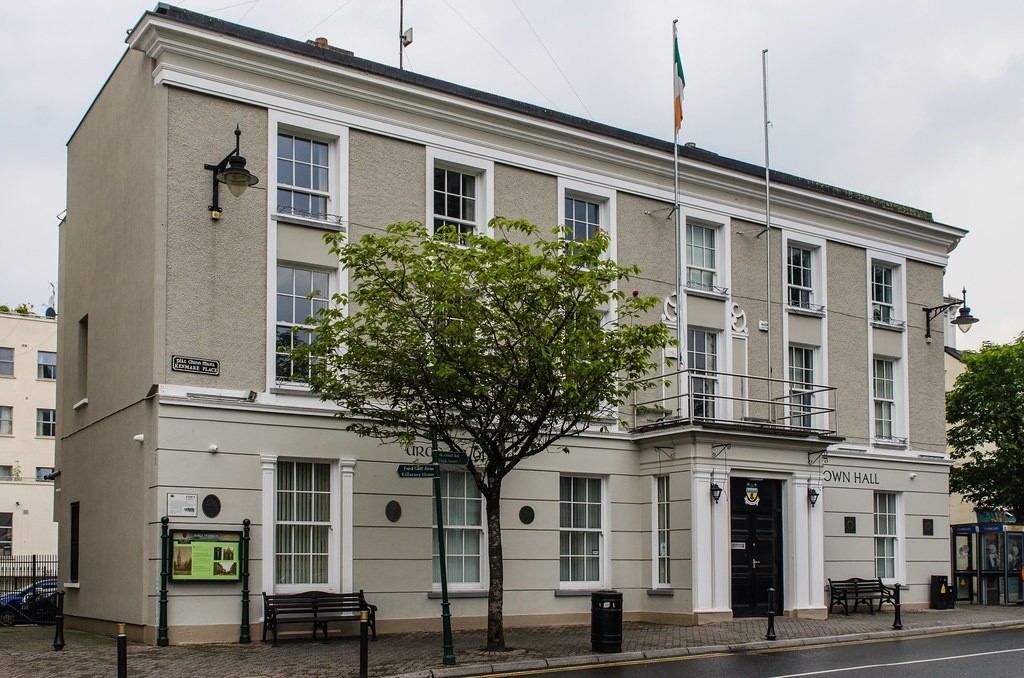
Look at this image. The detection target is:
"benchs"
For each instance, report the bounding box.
[263,589,376,646]
[827,577,897,617]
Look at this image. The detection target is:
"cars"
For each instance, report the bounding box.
[22,584,58,626]
[1,579,59,627]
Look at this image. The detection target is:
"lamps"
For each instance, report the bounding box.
[710,482,723,504]
[808,488,819,507]
[921,285,982,344]
[204,122,260,219]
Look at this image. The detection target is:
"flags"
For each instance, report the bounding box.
[675,25,687,137]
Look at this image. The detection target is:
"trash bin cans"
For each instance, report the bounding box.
[930,575,948,611]
[591,589,624,653]
